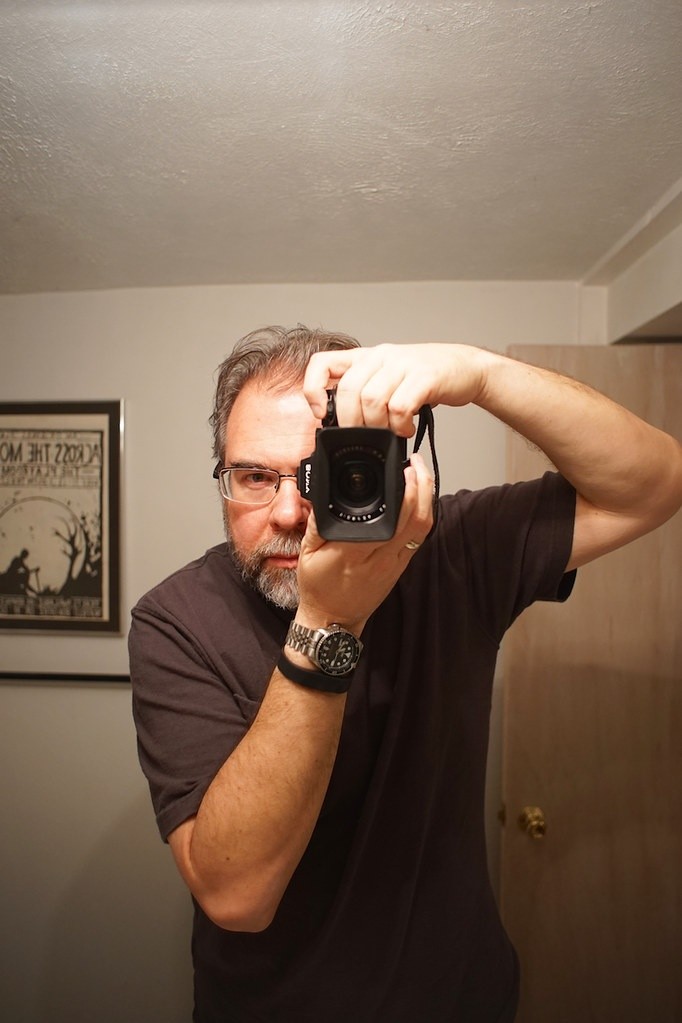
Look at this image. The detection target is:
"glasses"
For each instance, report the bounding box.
[213,460,300,505]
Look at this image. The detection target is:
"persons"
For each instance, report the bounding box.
[125,327,682,1023]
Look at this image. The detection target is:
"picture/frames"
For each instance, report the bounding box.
[0,398,126,636]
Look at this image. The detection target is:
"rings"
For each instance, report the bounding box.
[405,540,420,550]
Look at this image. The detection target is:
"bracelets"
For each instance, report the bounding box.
[277,649,352,694]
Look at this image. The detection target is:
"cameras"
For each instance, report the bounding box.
[296,387,411,542]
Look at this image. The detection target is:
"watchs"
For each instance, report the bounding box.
[286,622,364,675]
